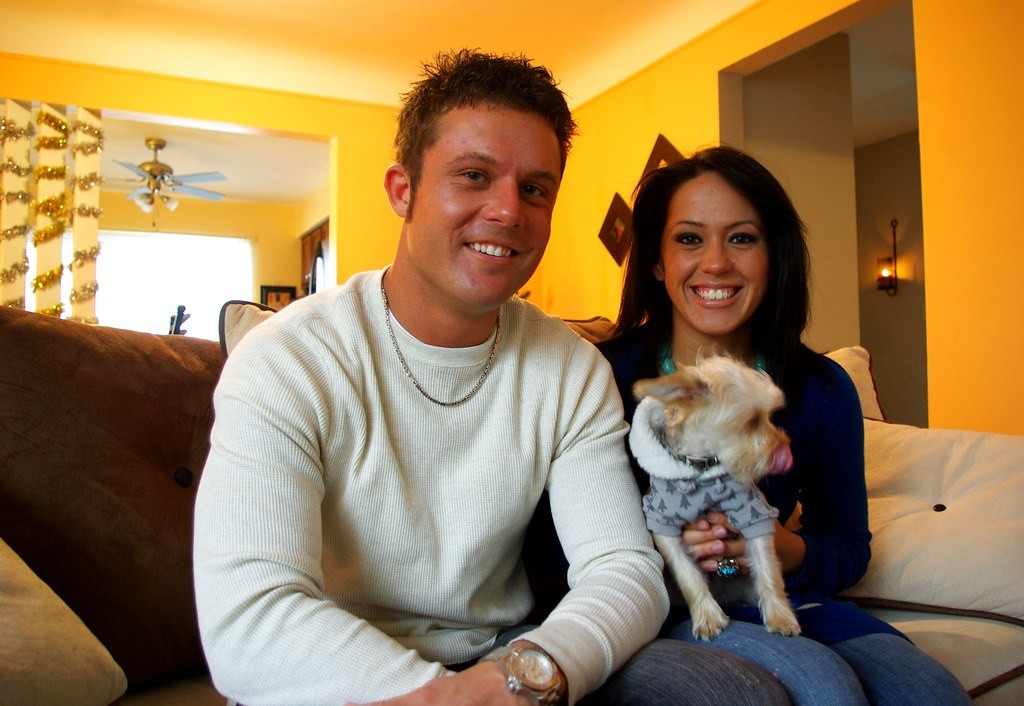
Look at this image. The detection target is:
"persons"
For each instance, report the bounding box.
[525,147,979,706]
[192,43,801,706]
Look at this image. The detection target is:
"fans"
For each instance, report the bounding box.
[103,137,226,228]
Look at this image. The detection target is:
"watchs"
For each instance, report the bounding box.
[477,639,570,706]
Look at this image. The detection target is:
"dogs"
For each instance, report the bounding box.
[626,358,802,642]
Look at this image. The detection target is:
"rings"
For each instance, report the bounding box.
[716,555,741,577]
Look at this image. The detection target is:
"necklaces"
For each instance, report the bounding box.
[656,346,768,378]
[380,279,501,405]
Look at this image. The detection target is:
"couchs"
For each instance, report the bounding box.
[0,306,1023,706]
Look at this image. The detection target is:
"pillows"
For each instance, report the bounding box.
[838,417,1024,629]
[220,300,277,365]
[823,343,885,422]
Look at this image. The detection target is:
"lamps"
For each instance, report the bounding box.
[876,219,899,296]
[133,180,177,213]
